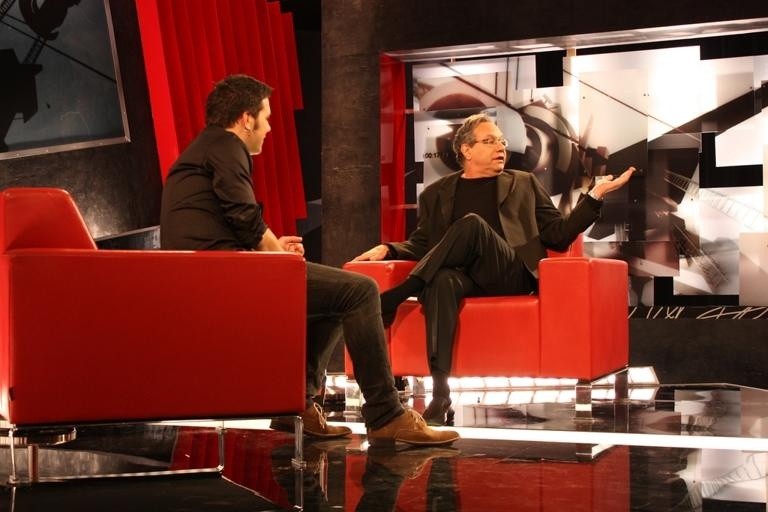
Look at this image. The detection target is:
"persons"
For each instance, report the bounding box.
[159,74,461,448]
[268,435,462,512]
[348,114,637,427]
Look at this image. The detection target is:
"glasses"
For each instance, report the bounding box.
[475,139,509,147]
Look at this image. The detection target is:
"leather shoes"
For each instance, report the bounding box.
[366,448,461,478]
[381,293,396,328]
[272,439,352,475]
[270,399,351,438]
[422,397,455,425]
[368,409,460,449]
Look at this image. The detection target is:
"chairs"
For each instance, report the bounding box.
[338,233,629,417]
[3,183,309,487]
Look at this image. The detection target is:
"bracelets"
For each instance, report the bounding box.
[588,190,605,201]
